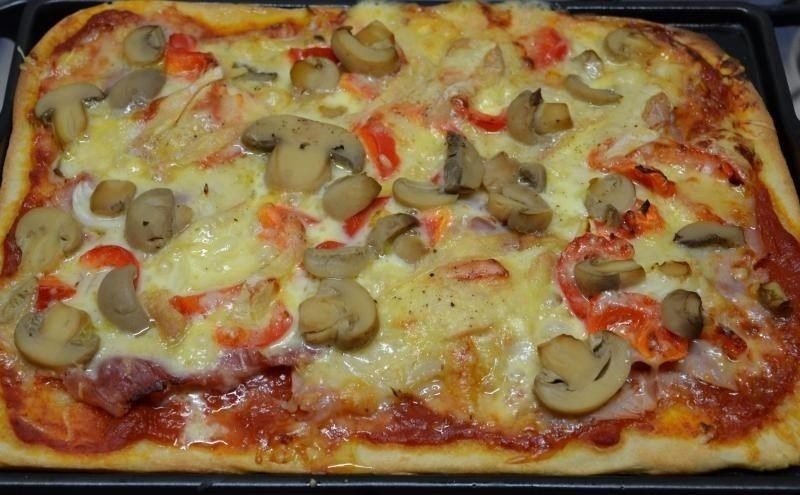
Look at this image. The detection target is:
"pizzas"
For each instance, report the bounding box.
[1,0,800,475]
